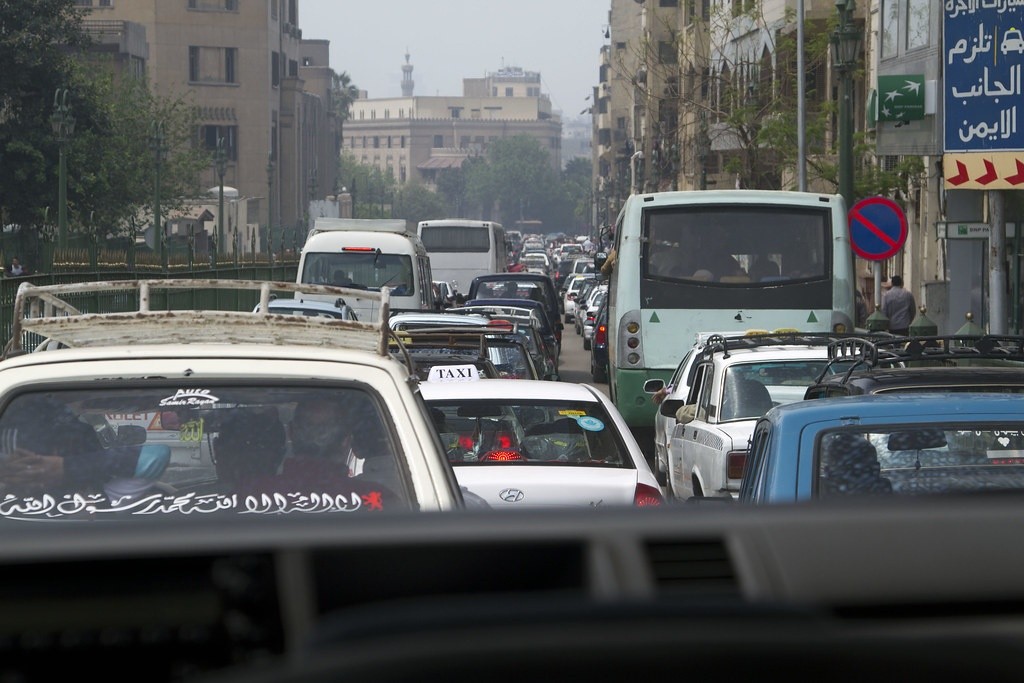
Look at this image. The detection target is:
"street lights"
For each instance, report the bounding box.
[827,0,860,287]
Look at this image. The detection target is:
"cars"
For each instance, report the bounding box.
[642,332,787,487]
[0,211,666,515]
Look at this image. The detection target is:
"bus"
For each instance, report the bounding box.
[594,189,856,451]
[418,220,514,297]
[659,335,893,502]
[293,228,435,324]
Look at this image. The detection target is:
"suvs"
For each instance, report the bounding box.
[736,335,1024,505]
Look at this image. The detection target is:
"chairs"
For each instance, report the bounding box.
[760,275,791,282]
[521,420,588,461]
[722,379,772,418]
[720,275,751,283]
[819,434,892,496]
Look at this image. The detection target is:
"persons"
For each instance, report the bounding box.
[600,251,615,274]
[883,277,916,336]
[333,270,345,286]
[7,258,27,301]
[856,291,867,328]
[238,394,401,509]
[335,298,358,321]
[501,283,518,297]
[826,436,880,510]
[652,387,672,403]
[685,228,739,280]
